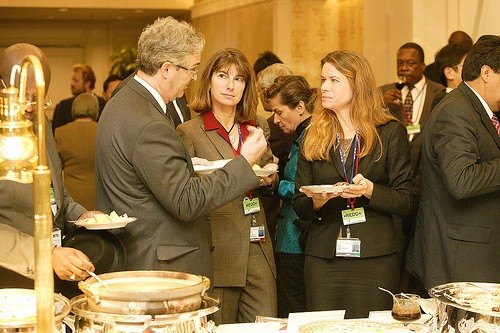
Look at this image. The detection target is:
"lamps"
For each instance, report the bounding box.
[0,55,55,333]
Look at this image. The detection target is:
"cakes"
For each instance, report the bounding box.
[80,211,129,223]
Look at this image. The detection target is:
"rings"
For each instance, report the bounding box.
[69,273,76,281]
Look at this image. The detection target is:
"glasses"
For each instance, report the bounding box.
[0,78,52,111]
[166,60,198,73]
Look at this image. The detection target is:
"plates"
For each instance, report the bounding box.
[254,170,279,176]
[369,310,400,322]
[298,320,397,333]
[75,217,137,230]
[302,185,348,193]
[286,310,346,333]
[194,159,233,175]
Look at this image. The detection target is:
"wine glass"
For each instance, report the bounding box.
[391,294,421,325]
[392,76,406,104]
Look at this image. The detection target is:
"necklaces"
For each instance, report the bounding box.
[341,130,366,226]
[280,143,292,209]
[229,121,265,242]
[401,81,428,134]
[341,132,355,158]
[336,127,361,257]
[221,120,237,143]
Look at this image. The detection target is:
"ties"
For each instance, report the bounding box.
[166,110,175,129]
[491,113,500,138]
[167,101,182,129]
[403,84,416,140]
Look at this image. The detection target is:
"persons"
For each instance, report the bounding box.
[103,48,192,131]
[264,75,317,319]
[96,15,267,297]
[0,43,112,289]
[52,64,106,138]
[293,50,416,319]
[176,47,277,322]
[54,93,99,211]
[404,35,500,299]
[378,31,474,164]
[254,51,297,246]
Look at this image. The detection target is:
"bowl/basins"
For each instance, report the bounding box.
[78,271,211,319]
[427,282,500,333]
